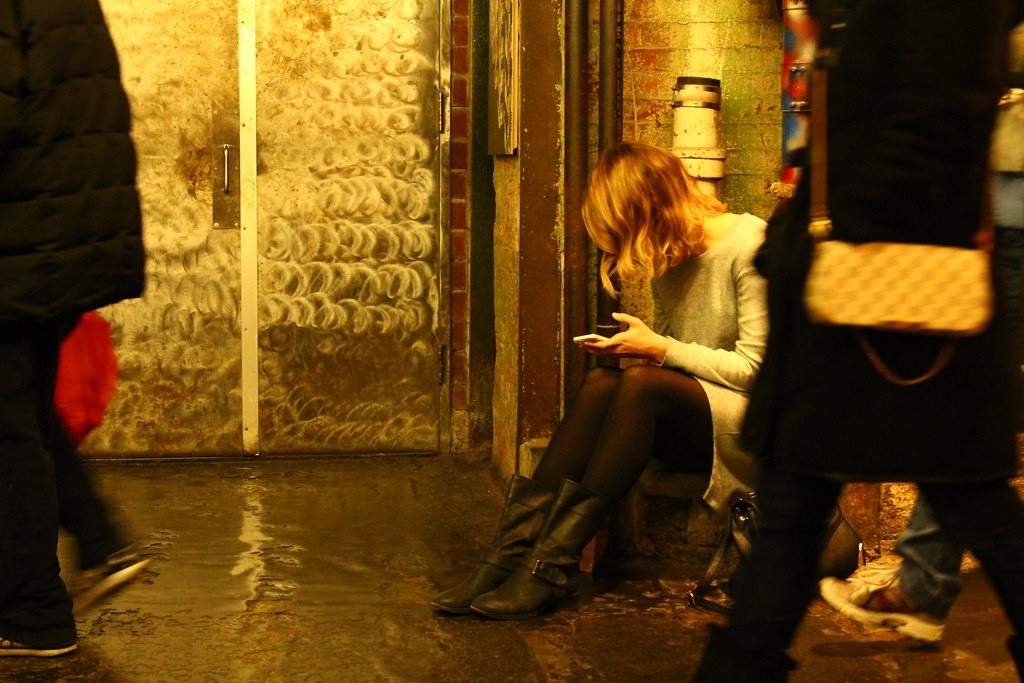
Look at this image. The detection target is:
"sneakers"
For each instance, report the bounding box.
[821,563,946,643]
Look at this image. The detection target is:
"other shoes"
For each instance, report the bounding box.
[1,637,77,658]
[76,546,149,609]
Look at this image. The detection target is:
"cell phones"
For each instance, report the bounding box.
[573,333,610,344]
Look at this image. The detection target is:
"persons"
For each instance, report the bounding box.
[0,0,149,656]
[693,1,1024,683]
[429,143,767,618]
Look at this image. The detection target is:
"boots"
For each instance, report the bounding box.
[431,473,560,614]
[470,477,617,619]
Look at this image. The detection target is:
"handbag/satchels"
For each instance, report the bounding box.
[731,491,869,581]
[779,238,1024,483]
